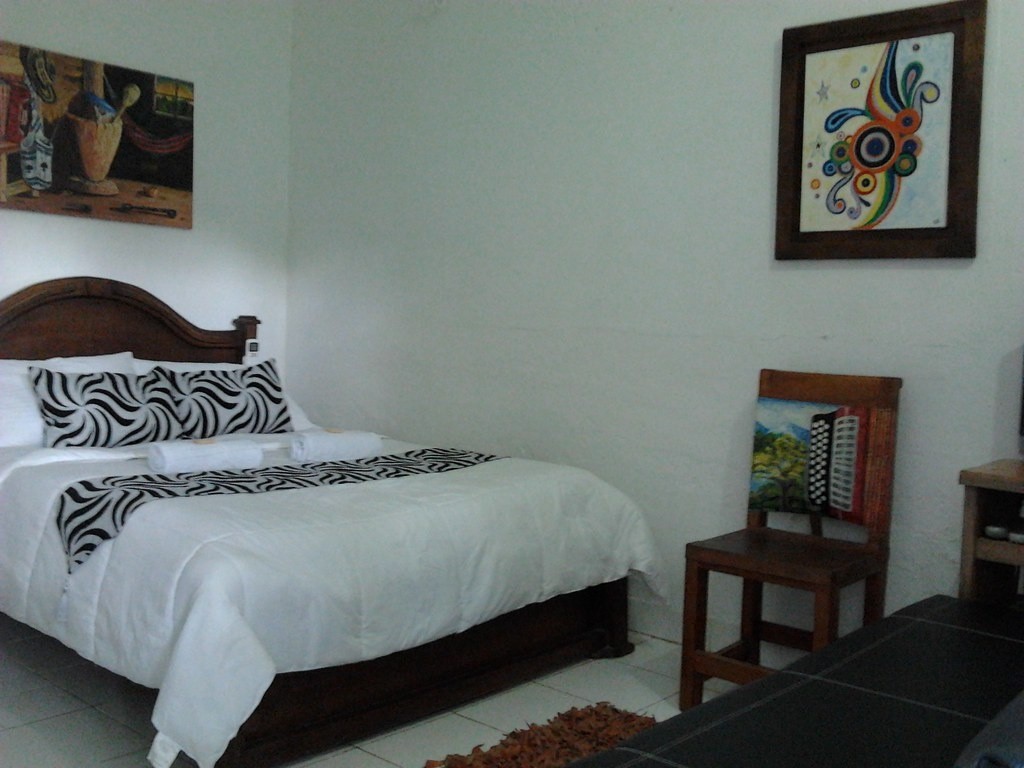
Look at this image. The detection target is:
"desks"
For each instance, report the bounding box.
[563,593,1024,768]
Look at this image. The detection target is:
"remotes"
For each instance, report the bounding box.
[245,340,259,358]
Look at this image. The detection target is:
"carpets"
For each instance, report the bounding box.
[422,699,659,768]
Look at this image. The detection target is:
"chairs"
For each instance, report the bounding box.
[679,368,904,713]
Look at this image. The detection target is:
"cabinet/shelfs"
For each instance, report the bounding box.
[956,456,1023,596]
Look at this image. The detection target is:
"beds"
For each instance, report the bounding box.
[0,276,668,768]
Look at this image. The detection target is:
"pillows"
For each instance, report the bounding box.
[167,357,297,440]
[134,347,321,433]
[0,349,134,448]
[28,366,186,448]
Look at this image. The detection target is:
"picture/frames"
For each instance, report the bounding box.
[775,1,988,261]
